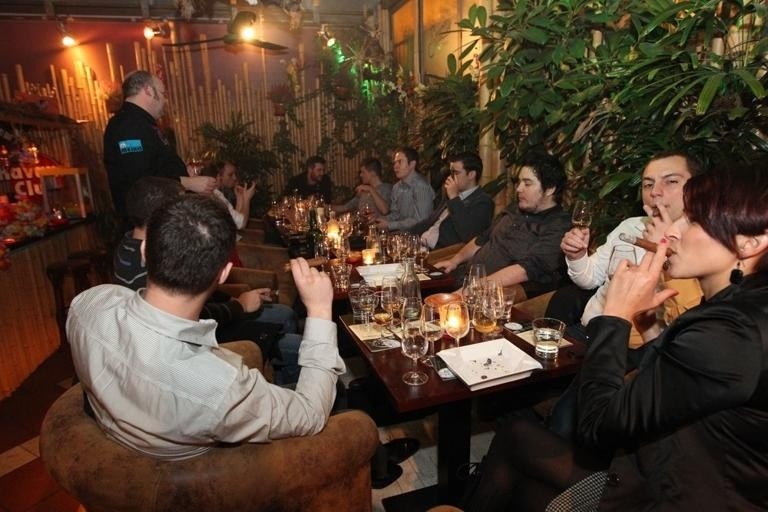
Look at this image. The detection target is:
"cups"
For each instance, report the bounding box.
[321,214,352,289]
[529,316,568,357]
[570,198,593,230]
[608,244,636,279]
[361,249,376,265]
[356,192,376,226]
[344,286,521,330]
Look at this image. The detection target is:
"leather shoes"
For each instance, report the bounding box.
[371,465,402,489]
[381,438,420,466]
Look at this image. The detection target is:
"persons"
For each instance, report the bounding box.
[328,158,393,219]
[284,157,331,203]
[409,151,495,251]
[65,194,346,462]
[209,160,257,229]
[559,149,704,353]
[101,70,217,220]
[434,151,575,295]
[460,166,767,512]
[375,147,435,230]
[112,177,306,383]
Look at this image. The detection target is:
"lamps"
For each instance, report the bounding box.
[58,17,76,48]
[143,25,170,40]
[316,23,336,50]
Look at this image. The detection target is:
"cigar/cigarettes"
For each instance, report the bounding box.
[618,230,674,258]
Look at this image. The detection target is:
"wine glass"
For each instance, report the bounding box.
[269,190,325,231]
[376,262,506,388]
[386,232,433,271]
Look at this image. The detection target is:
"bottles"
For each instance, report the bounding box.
[306,210,321,249]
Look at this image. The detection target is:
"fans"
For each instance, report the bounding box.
[160,21,287,52]
